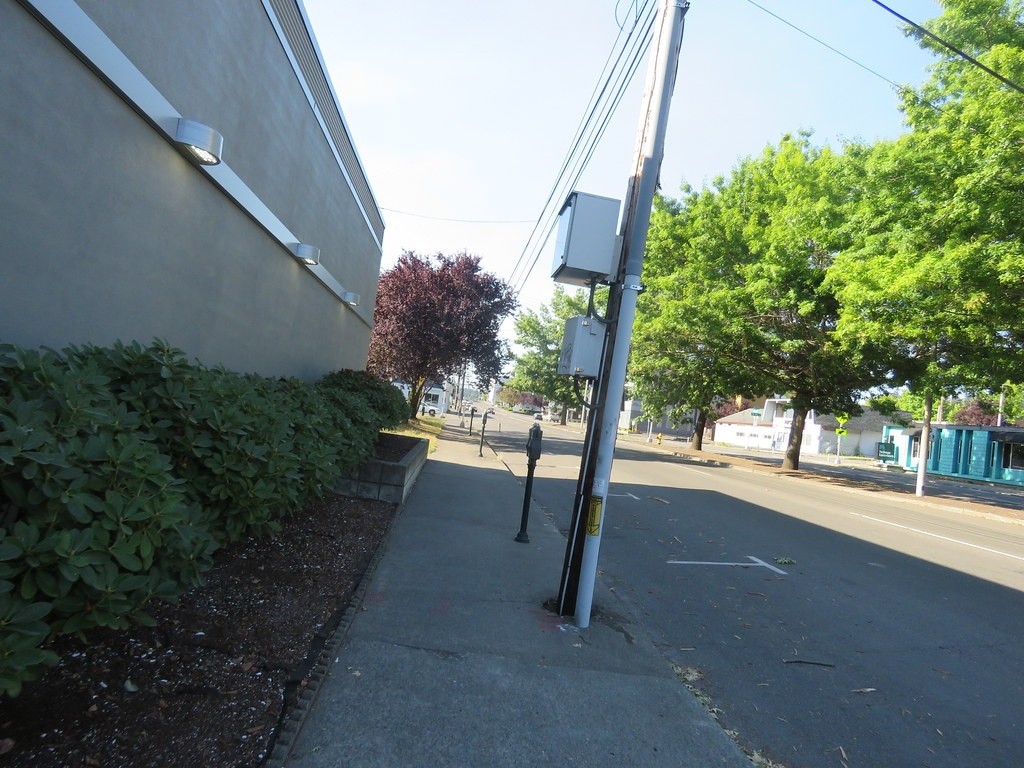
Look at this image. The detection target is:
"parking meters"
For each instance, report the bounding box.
[478,410,488,457]
[469,406,475,436]
[514,422,543,544]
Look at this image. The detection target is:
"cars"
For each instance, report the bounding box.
[533,413,543,420]
[546,412,560,423]
[487,407,495,415]
[462,400,477,412]
[418,401,440,415]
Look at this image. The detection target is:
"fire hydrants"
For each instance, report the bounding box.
[656,432,662,445]
